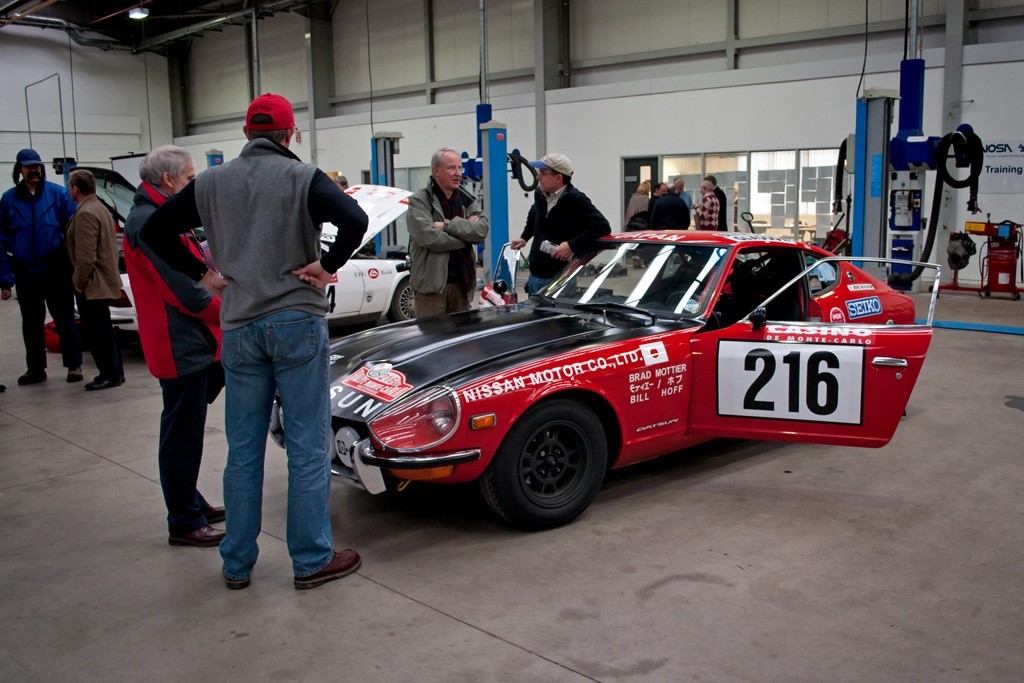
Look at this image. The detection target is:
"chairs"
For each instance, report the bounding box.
[637,258,803,326]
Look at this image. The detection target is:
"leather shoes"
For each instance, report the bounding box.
[207,504,226,522]
[168,525,227,547]
[221,566,251,590]
[293,547,363,590]
[84,376,126,390]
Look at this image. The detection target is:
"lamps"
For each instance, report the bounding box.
[129,7,149,20]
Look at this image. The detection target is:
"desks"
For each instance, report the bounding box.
[784,223,816,244]
[751,222,772,234]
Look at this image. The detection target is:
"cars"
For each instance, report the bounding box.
[61,163,416,354]
[272,226,945,534]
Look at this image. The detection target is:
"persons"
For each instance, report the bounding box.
[621,175,729,232]
[120,146,231,550]
[405,149,491,317]
[139,94,369,593]
[509,151,612,299]
[0,149,87,386]
[333,175,349,192]
[61,167,127,393]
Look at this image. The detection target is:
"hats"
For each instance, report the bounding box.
[527,152,574,177]
[16,149,43,166]
[244,93,295,131]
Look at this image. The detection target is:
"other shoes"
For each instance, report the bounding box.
[66,368,83,382]
[18,370,47,384]
